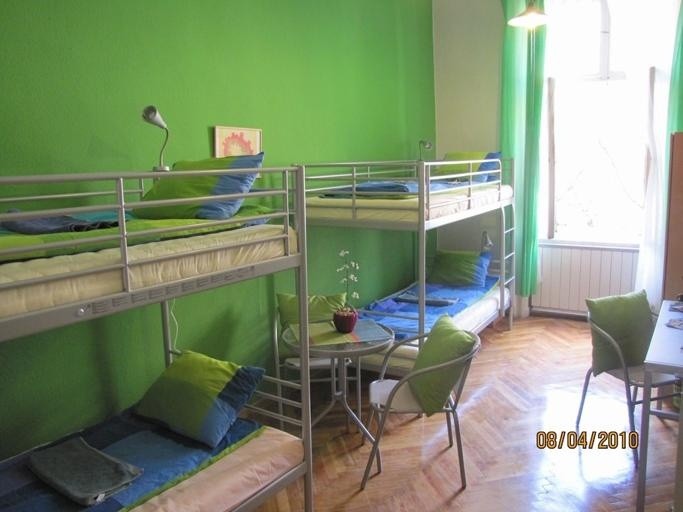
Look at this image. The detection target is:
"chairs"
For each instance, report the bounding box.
[359,328,481,490]
[576,309,663,470]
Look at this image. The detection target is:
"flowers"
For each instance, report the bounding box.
[335,250,360,312]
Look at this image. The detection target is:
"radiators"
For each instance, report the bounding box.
[529,238,641,319]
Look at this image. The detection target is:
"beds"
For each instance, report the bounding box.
[0,164,313,511]
[257,158,517,417]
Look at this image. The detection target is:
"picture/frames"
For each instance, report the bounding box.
[213,125,262,179]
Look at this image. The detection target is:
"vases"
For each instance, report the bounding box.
[333,309,358,333]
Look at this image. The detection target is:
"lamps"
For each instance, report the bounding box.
[419,140,432,161]
[507,0,550,30]
[481,230,493,251]
[142,105,170,172]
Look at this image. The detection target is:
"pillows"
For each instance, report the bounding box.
[432,151,503,182]
[428,247,492,288]
[584,288,655,377]
[132,151,264,220]
[408,312,476,417]
[133,349,266,450]
[273,291,348,359]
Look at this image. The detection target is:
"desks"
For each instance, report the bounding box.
[281,318,395,474]
[634,299,683,512]
